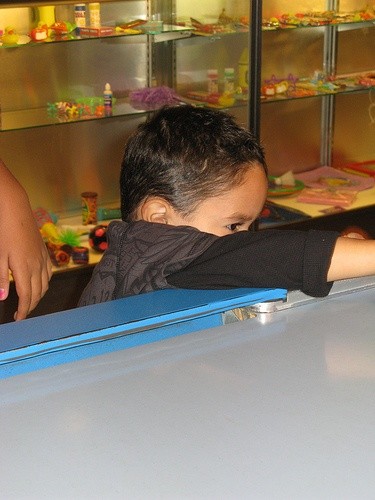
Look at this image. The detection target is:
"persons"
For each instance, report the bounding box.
[74,104,375,308]
[0,160,53,323]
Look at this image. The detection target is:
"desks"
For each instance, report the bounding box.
[0,275,375,500]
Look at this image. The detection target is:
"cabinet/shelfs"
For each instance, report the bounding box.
[0,0,375,324]
